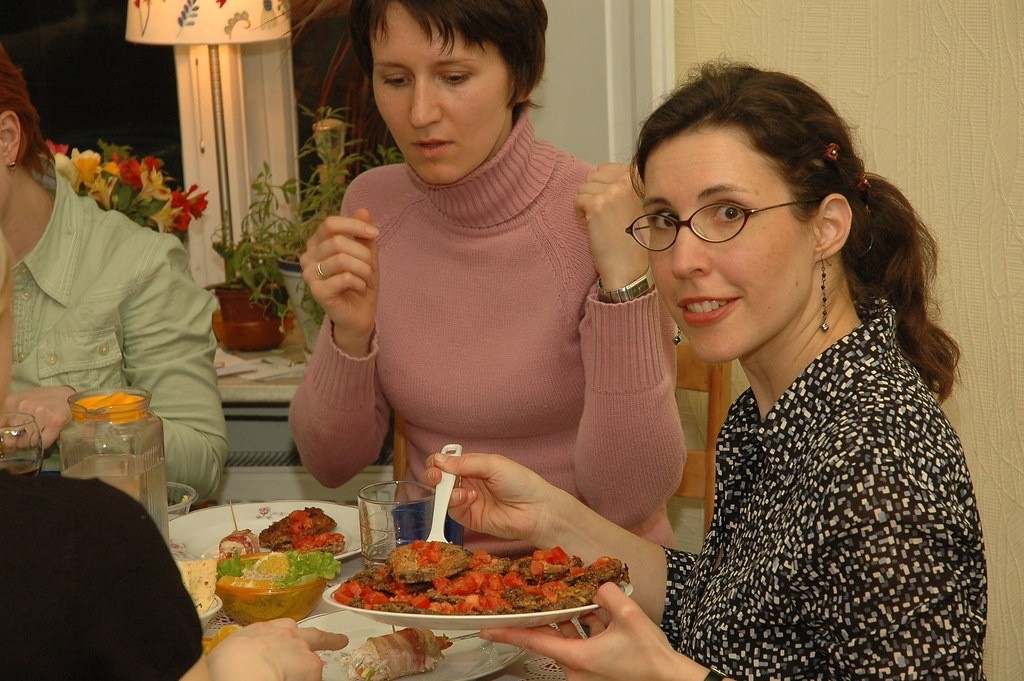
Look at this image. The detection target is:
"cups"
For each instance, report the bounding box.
[0,412,43,476]
[357,481,436,569]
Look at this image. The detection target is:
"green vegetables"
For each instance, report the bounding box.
[218,551,342,589]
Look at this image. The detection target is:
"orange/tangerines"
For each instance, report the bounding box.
[256,556,289,576]
[217,574,273,589]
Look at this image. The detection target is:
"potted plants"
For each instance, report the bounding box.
[208,103,403,351]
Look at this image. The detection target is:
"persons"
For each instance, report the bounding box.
[422,52,988,681]
[289,0,688,559]
[0,467,350,681]
[0,42,230,510]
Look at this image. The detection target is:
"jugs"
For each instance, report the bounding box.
[60,386,169,544]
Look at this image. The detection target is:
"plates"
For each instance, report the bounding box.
[296,609,529,681]
[322,583,634,629]
[169,500,388,561]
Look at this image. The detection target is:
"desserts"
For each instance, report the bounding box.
[219,530,259,556]
[340,627,453,681]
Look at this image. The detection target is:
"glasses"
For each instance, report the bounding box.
[623,200,825,254]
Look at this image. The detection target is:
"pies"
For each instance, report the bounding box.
[176,559,217,616]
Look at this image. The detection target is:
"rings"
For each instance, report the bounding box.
[315,263,327,280]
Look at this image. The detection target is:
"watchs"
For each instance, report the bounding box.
[596,261,656,304]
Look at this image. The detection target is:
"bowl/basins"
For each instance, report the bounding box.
[167,482,198,521]
[199,595,222,630]
[215,553,328,626]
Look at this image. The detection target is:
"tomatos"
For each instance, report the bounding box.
[334,539,585,612]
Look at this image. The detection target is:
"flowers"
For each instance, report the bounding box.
[46,140,210,236]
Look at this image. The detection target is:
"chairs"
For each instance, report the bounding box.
[668,342,733,540]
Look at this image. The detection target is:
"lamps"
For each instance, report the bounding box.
[124,0,294,279]
